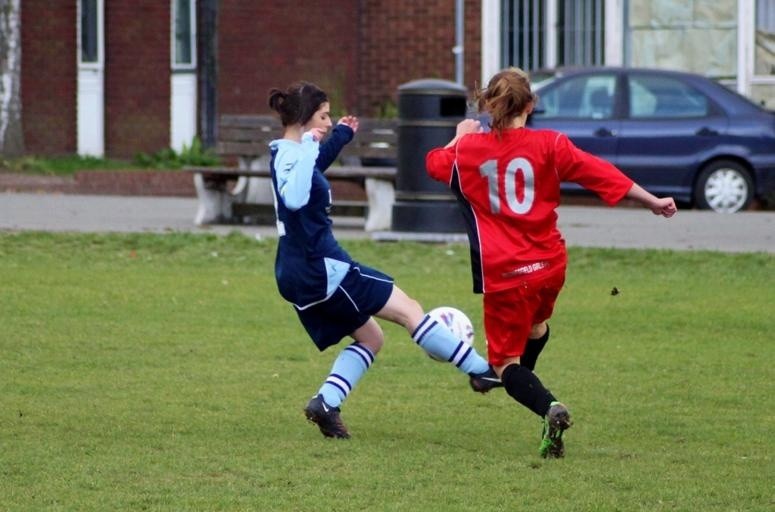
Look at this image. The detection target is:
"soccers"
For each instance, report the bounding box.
[423,307,475,361]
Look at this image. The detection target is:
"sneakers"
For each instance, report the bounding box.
[304,392,353,441]
[469,363,505,394]
[536,401,573,460]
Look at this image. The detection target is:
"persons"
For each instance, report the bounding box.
[424,65,679,463]
[265,79,507,439]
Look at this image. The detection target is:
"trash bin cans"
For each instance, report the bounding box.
[392,78,468,233]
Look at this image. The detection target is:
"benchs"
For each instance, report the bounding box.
[182,164,397,230]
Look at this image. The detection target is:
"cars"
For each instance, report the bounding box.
[338,61,774,214]
